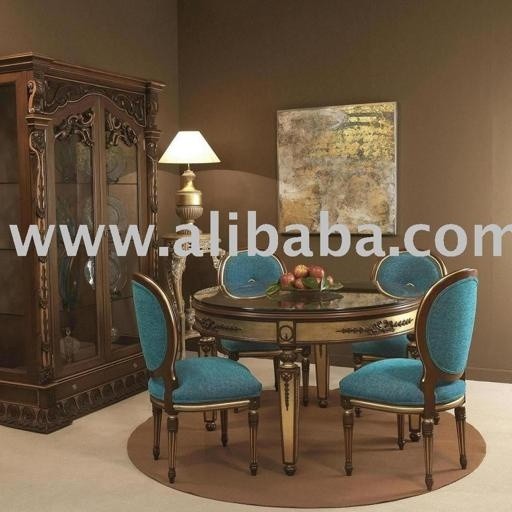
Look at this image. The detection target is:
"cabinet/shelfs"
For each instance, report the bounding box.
[1,50,169,434]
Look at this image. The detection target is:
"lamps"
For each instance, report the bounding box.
[156,130,222,235]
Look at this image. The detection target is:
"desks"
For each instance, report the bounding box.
[191,286,423,476]
[151,234,227,360]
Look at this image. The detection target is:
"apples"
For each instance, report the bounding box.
[327,275,334,286]
[280,272,296,289]
[293,264,309,278]
[316,277,322,287]
[295,277,306,289]
[309,264,325,278]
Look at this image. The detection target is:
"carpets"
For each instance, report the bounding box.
[125,384,488,510]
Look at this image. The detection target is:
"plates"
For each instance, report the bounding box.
[83,252,128,292]
[273,292,344,302]
[81,195,127,234]
[78,144,127,180]
[280,284,343,291]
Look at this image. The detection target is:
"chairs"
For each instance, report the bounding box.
[130,272,264,484]
[338,266,481,491]
[215,250,311,414]
[351,249,448,430]
